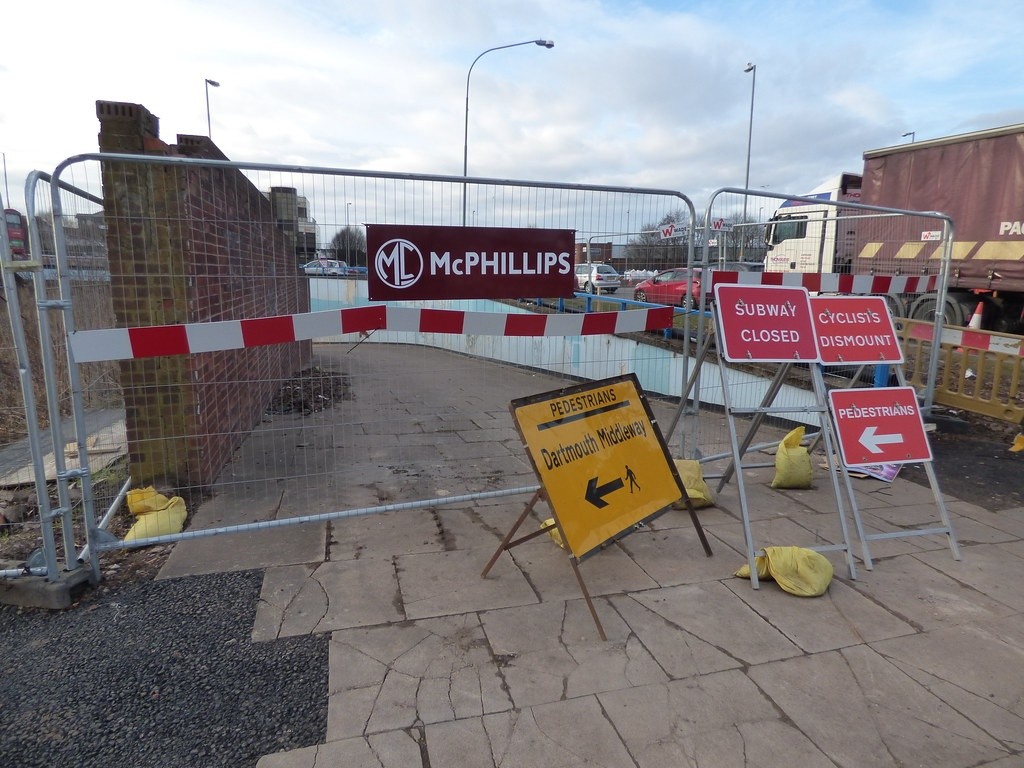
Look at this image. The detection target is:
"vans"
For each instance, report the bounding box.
[704,261,766,273]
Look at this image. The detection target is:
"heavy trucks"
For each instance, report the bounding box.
[758,122,1024,348]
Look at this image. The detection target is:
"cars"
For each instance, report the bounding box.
[633,266,703,310]
[574,263,621,294]
[303,257,370,278]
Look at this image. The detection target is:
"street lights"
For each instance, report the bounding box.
[737,61,758,260]
[204,77,221,139]
[459,37,554,226]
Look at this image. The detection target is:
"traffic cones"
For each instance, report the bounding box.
[954,301,986,355]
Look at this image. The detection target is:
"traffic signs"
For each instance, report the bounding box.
[714,283,821,364]
[807,295,906,366]
[509,371,688,566]
[828,386,936,469]
